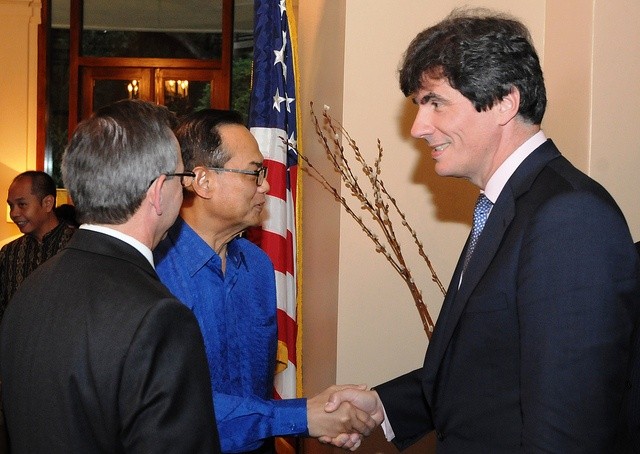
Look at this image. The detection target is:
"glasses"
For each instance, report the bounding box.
[145,170,196,198]
[207,166,268,186]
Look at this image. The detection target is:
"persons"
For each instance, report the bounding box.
[316,8,640,454]
[0,172,75,313]
[156,107,377,453]
[0,99,223,453]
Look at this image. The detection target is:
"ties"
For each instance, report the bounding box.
[463,194,492,284]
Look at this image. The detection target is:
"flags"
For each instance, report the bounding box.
[246,0,303,454]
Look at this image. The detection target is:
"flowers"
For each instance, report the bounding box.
[279,101,447,343]
